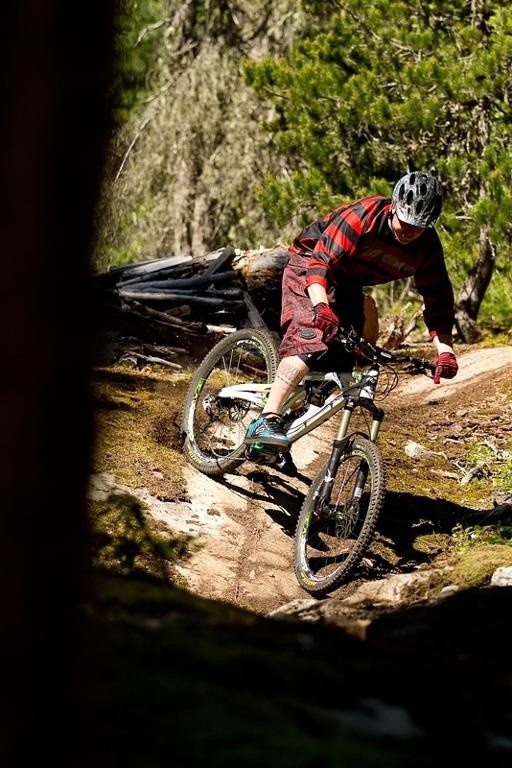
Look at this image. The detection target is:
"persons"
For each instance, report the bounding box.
[243,171,459,446]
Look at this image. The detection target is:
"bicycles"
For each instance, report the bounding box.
[179,315,438,594]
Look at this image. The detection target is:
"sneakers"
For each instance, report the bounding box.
[268,450,297,476]
[242,414,292,453]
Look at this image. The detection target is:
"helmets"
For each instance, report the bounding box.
[391,170,445,228]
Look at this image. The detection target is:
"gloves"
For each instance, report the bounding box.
[311,302,341,344]
[432,352,459,384]
